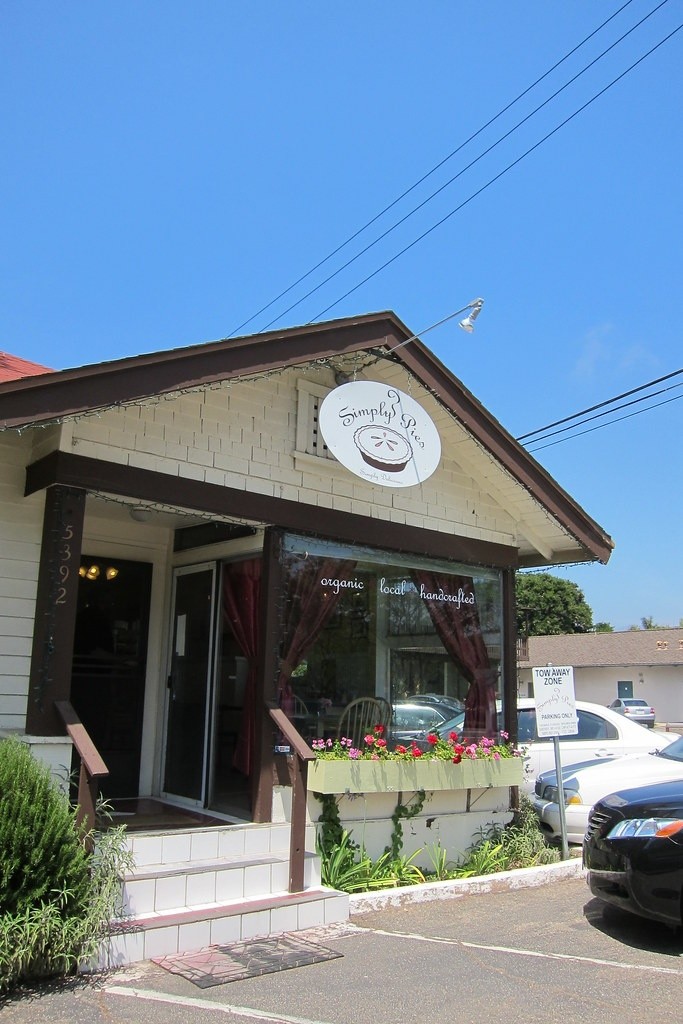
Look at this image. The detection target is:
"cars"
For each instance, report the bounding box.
[386,691,683,807]
[580,779,683,934]
[531,735,683,847]
[605,697,655,729]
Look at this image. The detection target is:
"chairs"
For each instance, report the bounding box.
[289,694,393,753]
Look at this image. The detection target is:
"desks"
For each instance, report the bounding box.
[290,713,373,741]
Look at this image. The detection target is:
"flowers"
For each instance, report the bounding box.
[316,697,332,711]
[311,726,536,785]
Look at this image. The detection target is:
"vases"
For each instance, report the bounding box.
[307,758,526,795]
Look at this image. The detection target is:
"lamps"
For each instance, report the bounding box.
[78,557,118,581]
[128,507,153,523]
[337,297,484,387]
